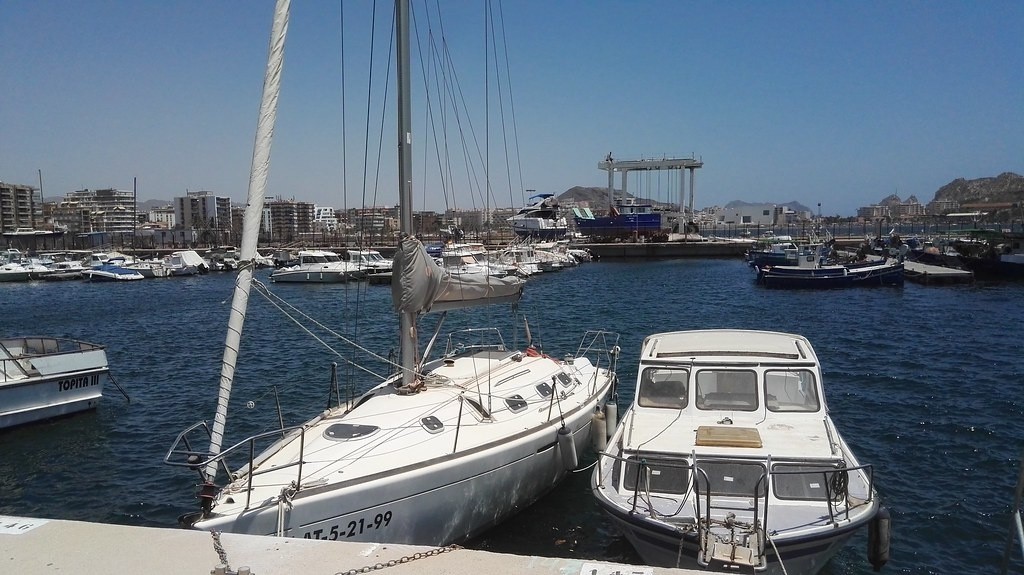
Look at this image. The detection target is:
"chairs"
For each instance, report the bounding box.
[651,381,685,403]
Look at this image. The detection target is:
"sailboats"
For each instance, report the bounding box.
[166,1,618,547]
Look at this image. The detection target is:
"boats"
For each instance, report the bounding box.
[745,221,1024,288]
[589,327,877,575]
[0,338,112,433]
[0,0,593,302]
